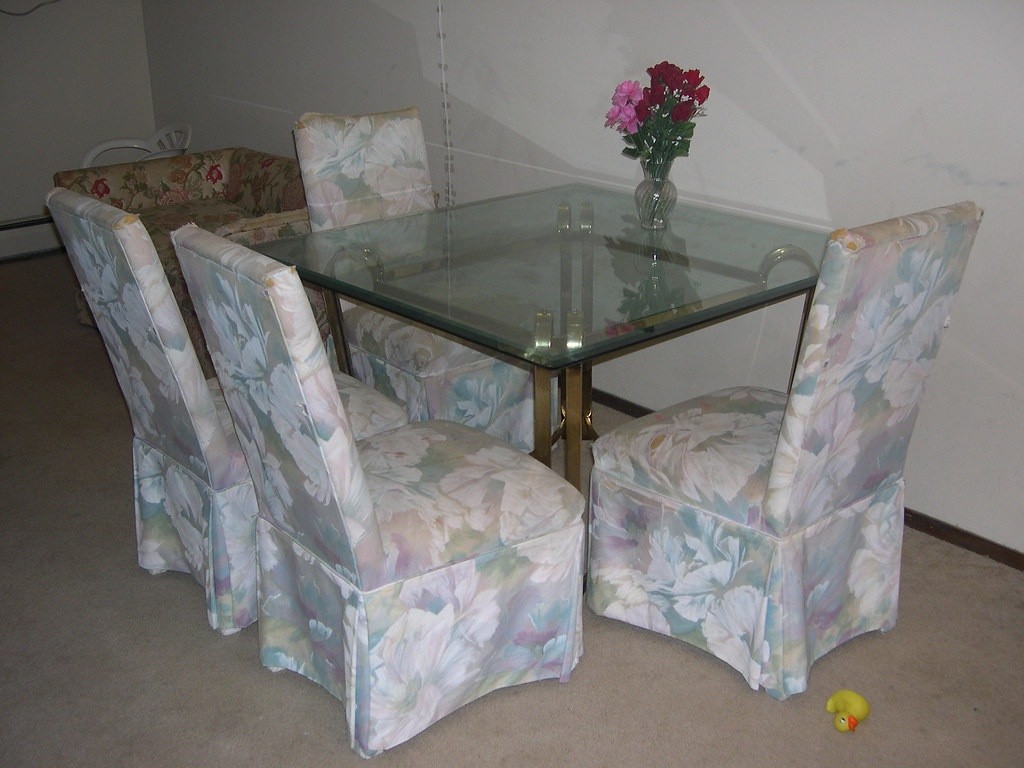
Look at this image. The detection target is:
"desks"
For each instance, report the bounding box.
[241,177,834,548]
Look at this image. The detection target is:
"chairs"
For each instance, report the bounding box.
[585,199,984,704]
[171,221,592,760]
[42,188,413,634]
[81,122,193,169]
[296,108,563,455]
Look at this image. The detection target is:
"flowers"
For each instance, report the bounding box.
[605,62,711,226]
[601,261,703,337]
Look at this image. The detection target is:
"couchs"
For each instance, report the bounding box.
[49,145,308,336]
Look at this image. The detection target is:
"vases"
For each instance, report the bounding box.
[631,222,676,277]
[634,158,676,231]
